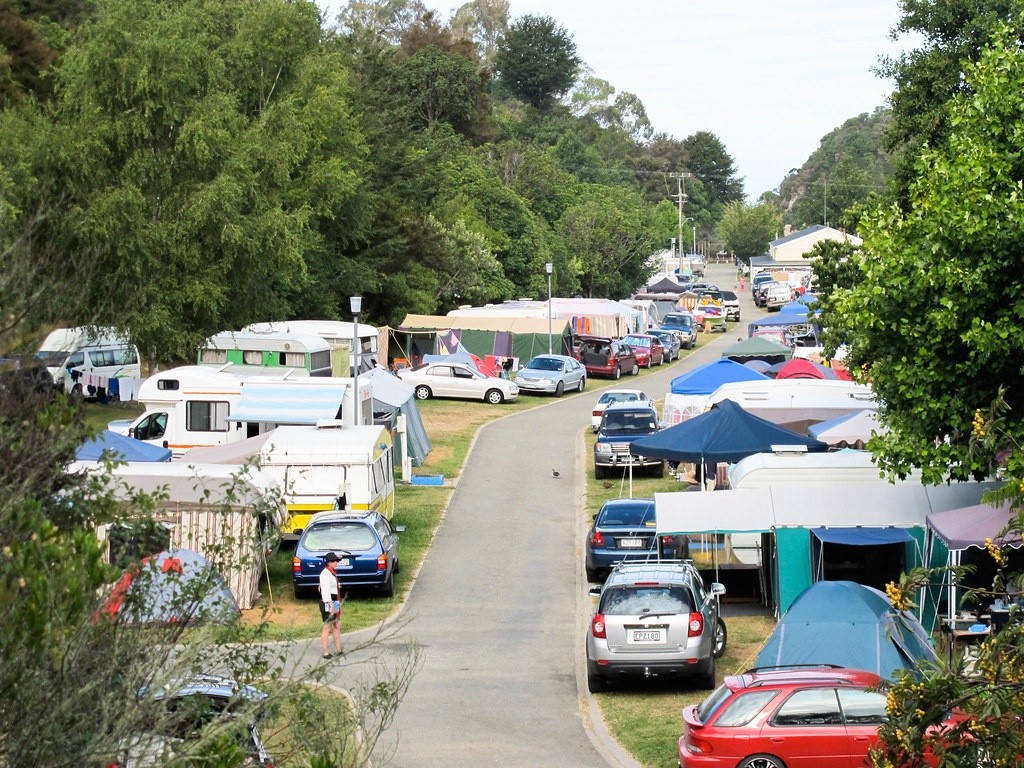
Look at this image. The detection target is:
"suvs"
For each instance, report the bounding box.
[583,562,735,691]
[677,668,1020,768]
[593,400,665,476]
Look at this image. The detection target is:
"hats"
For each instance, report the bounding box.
[326,552,340,562]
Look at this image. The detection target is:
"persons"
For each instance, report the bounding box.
[317,553,347,659]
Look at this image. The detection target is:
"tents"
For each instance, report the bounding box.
[751,580,957,707]
[347,367,432,467]
[401,314,574,374]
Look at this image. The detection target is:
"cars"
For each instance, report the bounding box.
[622,333,664,365]
[657,313,698,348]
[644,330,681,361]
[591,389,656,433]
[680,255,806,332]
[398,362,517,405]
[584,498,690,580]
[107,675,274,767]
[509,354,587,397]
[290,509,407,597]
[575,336,638,379]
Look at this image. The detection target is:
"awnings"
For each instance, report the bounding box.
[547,271,875,395]
[68,430,171,466]
[223,382,348,444]
[923,495,1024,664]
[807,409,892,447]
[628,403,819,565]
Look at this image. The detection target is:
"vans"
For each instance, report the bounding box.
[35,324,140,397]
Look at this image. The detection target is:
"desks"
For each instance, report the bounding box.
[991,601,1024,634]
[935,612,989,666]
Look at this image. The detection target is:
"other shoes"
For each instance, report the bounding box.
[323,652,343,659]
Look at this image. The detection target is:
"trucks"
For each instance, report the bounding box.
[110,320,393,545]
